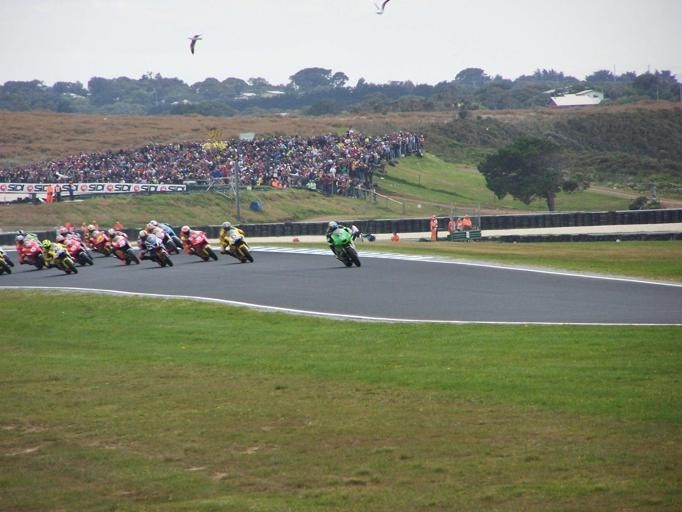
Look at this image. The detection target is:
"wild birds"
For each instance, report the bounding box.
[187,35,203,54]
[375,0,389,14]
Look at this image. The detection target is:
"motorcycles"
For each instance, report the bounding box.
[1,222,254,275]
[327,226,361,267]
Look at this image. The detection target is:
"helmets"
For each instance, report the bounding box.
[221,222,231,229]
[15,223,192,249]
[326,221,338,231]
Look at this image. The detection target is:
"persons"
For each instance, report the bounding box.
[1,139,228,202]
[429,214,439,241]
[455,217,464,232]
[463,214,473,230]
[447,217,456,233]
[390,231,400,241]
[326,220,353,259]
[223,125,426,199]
[351,223,360,241]
[12,218,249,269]
